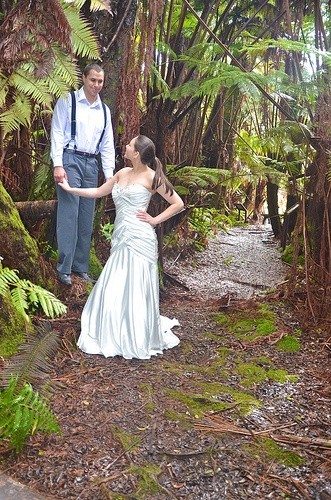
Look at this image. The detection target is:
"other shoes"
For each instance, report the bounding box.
[79,272,97,284]
[58,272,72,286]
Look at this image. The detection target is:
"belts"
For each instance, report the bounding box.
[64,149,96,158]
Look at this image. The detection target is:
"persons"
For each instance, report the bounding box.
[58,135,185,359]
[49,64,116,288]
